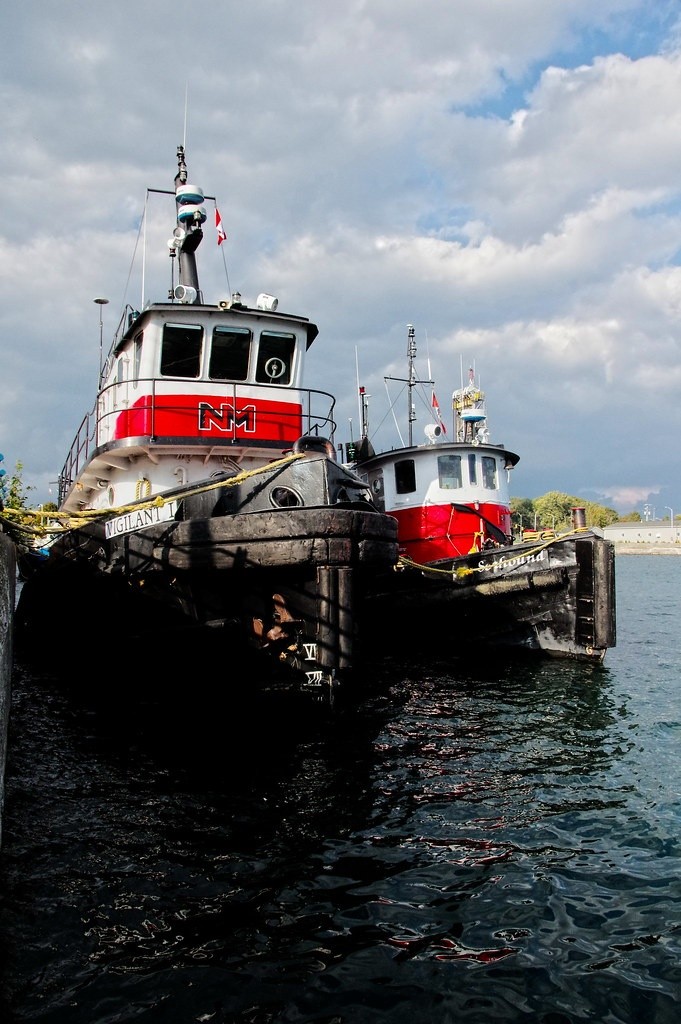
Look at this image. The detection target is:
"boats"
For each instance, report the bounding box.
[338,323,618,667]
[30,82,402,704]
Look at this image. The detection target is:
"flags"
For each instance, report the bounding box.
[432,392,447,434]
[215,208,226,245]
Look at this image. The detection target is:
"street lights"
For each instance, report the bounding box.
[548,514,555,529]
[664,506,675,528]
